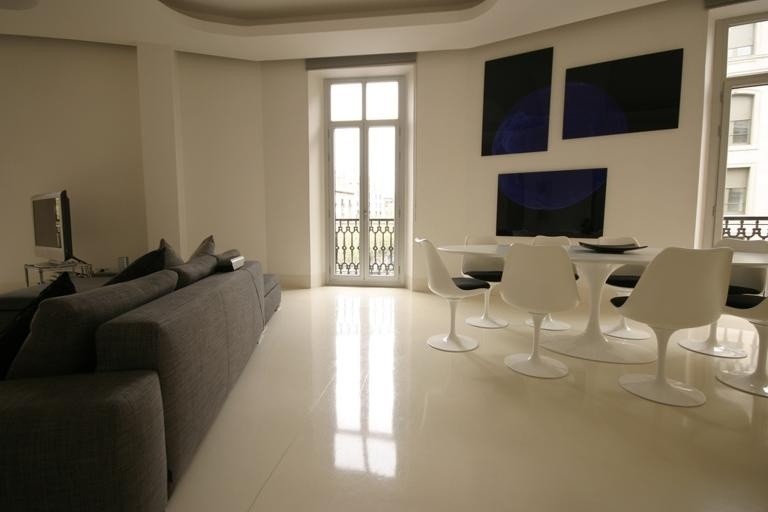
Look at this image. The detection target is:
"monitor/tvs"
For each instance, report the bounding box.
[494,166,609,239]
[29,188,76,269]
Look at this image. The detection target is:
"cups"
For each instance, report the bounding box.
[80,264,91,280]
[118,256,128,273]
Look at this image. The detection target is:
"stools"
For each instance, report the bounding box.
[0,277,113,337]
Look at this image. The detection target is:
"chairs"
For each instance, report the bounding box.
[610,247,732,408]
[676,238,768,358]
[716,290,767,399]
[415,237,490,351]
[598,235,651,341]
[499,243,579,380]
[462,234,508,328]
[525,234,571,331]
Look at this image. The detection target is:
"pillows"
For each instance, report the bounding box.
[184,236,217,263]
[160,239,183,268]
[104,249,165,286]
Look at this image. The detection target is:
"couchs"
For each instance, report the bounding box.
[0,249,281,512]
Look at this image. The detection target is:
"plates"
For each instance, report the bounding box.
[578,241,648,254]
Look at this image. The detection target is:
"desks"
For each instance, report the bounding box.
[23,261,81,288]
[437,246,768,363]
